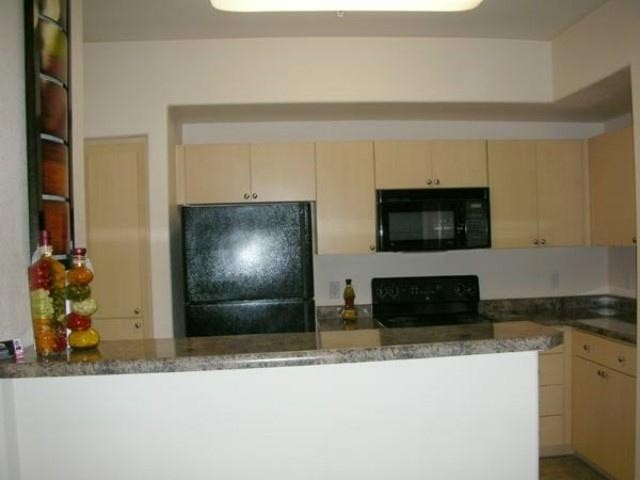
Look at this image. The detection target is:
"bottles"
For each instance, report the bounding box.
[67,248,101,350]
[344,278,355,309]
[27,229,68,355]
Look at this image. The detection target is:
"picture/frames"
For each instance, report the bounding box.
[24,0,76,275]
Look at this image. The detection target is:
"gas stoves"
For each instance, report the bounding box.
[372,312,488,329]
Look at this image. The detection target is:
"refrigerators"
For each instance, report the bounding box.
[184,203,314,332]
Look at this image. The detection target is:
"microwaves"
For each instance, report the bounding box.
[376,189,492,252]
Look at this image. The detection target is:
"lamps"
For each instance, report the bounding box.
[209,1,483,12]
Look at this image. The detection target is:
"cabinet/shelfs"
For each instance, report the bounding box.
[494,322,570,457]
[84,135,153,341]
[571,328,637,480]
